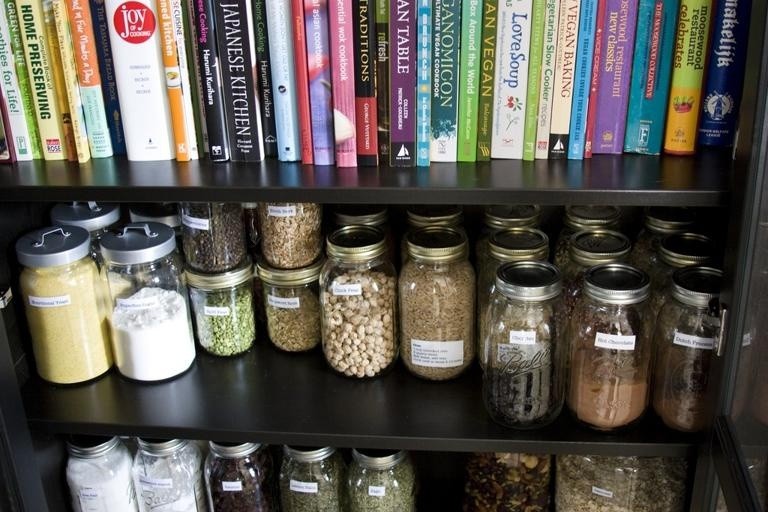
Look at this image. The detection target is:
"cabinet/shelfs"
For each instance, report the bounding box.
[0,0,768,512]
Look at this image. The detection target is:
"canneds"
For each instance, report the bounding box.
[318,204,399,379]
[633,210,724,427]
[65,435,139,510]
[181,201,256,357]
[482,208,571,428]
[256,202,327,353]
[555,208,656,427]
[348,448,412,510]
[397,205,473,381]
[132,438,207,510]
[279,445,349,510]
[203,441,279,511]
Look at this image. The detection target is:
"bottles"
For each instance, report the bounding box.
[58,433,695,512]
[15,202,731,431]
